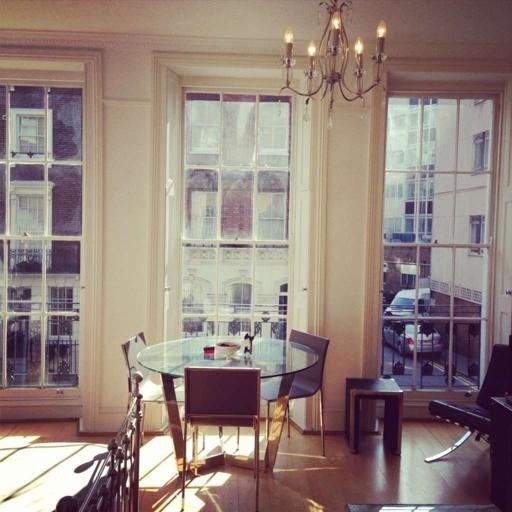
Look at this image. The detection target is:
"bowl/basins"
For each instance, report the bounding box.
[215,341,241,352]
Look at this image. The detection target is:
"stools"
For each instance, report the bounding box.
[346,378,403,455]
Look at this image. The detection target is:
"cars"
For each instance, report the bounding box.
[383,282,443,357]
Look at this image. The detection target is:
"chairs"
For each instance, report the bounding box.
[424,344,512,462]
[121,331,198,468]
[181,366,260,511]
[237,329,330,456]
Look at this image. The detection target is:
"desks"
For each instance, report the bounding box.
[490,397,512,512]
[137,336,320,480]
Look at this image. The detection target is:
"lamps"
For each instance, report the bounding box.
[277,0,390,129]
